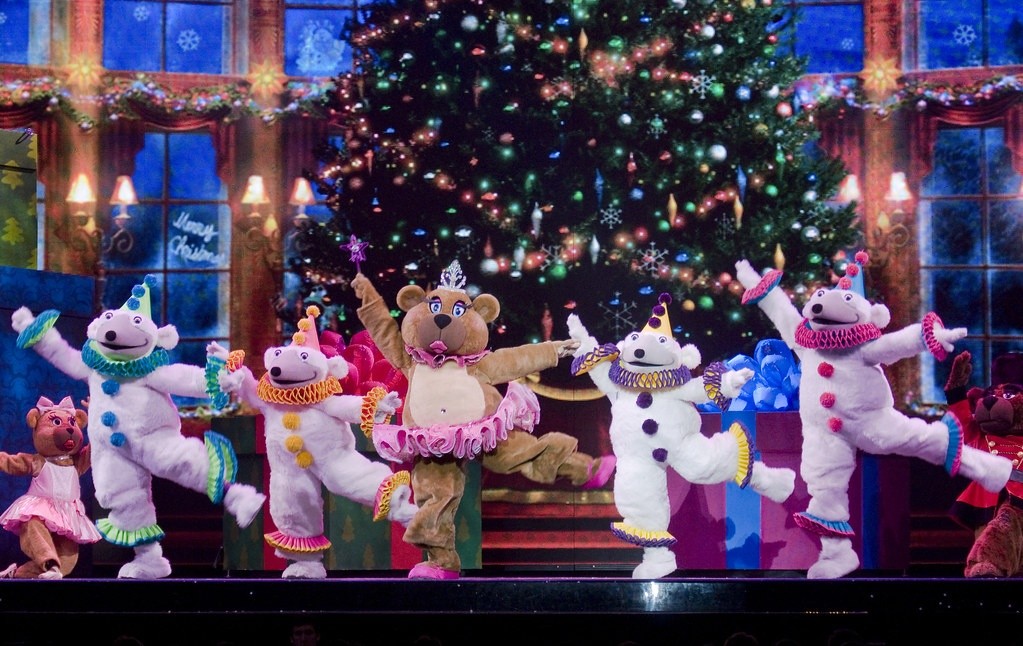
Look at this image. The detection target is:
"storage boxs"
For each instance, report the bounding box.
[213,414,484,574]
[165,406,225,525]
[478,364,619,505]
[665,408,881,573]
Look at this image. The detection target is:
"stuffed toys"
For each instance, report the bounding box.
[0,255,1023,585]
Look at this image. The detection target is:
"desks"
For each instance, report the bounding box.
[0,576,1023,645]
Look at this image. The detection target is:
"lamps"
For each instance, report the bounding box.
[838,175,864,217]
[109,175,138,219]
[239,173,270,219]
[65,174,99,217]
[288,176,316,225]
[884,171,913,226]
